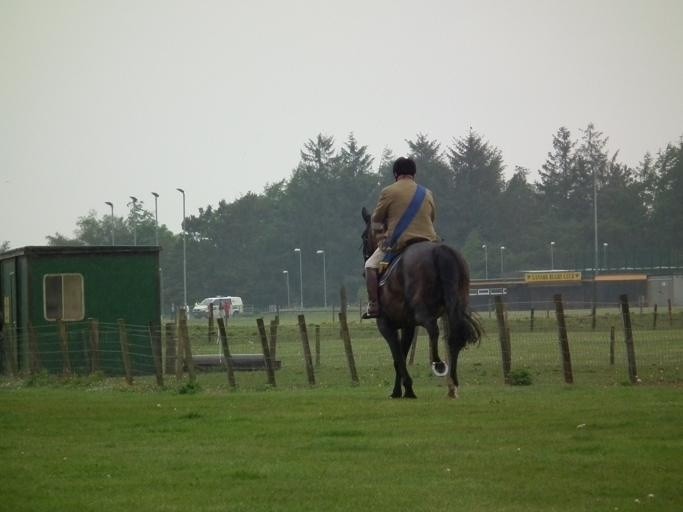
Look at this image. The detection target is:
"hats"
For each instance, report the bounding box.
[392,156,416,175]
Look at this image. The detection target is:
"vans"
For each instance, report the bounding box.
[192,295,243,320]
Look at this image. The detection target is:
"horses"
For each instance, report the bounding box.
[361,206,488,399]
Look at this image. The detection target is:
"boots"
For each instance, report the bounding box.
[364,267,380,317]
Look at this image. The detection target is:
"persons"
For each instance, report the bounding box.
[360,157,439,320]
[169,297,233,324]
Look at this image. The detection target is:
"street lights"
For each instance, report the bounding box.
[500,246,505,273]
[104,196,137,246]
[482,245,488,279]
[594,182,601,266]
[151,191,159,245]
[282,248,327,307]
[603,242,608,265]
[176,187,187,308]
[550,242,555,270]
